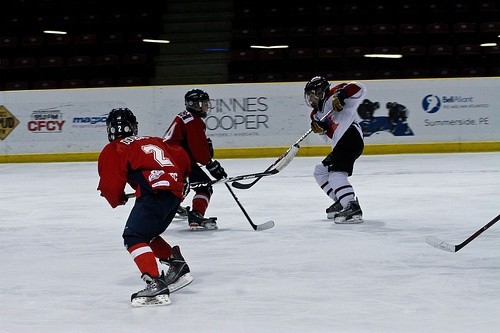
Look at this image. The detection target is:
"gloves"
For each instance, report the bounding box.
[207,137,214,158]
[206,160,227,180]
[311,120,330,134]
[122,195,127,205]
[331,95,344,112]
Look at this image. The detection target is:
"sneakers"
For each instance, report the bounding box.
[173,205,189,219]
[132,271,172,307]
[159,246,194,293]
[325,202,344,219]
[188,212,218,231]
[334,197,364,224]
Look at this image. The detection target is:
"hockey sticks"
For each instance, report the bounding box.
[426,214,500,252]
[125,143,301,199]
[231,108,336,189]
[213,160,275,231]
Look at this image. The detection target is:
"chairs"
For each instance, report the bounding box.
[0,0,169,90]
[229,0,500,82]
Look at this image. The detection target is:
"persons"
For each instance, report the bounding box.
[162,88,227,230]
[97,108,193,307]
[304,76,366,223]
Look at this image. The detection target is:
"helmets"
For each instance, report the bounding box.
[106,108,138,142]
[304,76,330,108]
[185,89,209,118]
